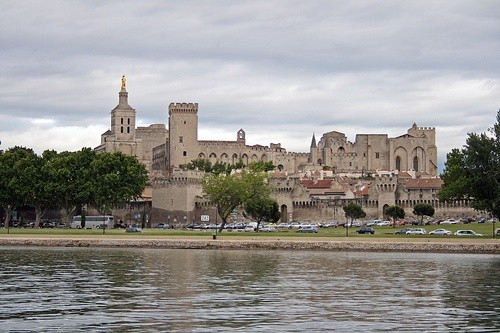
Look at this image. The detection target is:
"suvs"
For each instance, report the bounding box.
[454,230,483,237]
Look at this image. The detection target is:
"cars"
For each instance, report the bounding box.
[9,220,66,229]
[126,223,143,233]
[154,223,169,228]
[428,229,453,236]
[356,227,375,235]
[172,218,498,232]
[394,228,411,235]
[494,227,500,237]
[406,227,427,235]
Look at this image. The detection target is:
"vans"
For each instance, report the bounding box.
[298,225,319,233]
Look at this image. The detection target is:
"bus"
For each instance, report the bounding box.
[71,216,114,229]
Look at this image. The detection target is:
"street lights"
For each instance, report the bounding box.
[102,203,106,235]
[7,205,10,234]
